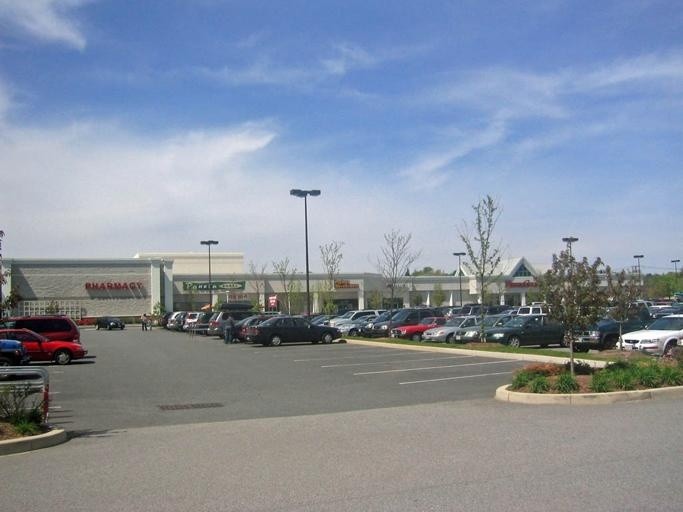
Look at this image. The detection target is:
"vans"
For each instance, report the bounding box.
[1,316,80,341]
[160,304,263,338]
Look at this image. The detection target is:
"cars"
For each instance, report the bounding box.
[0,340,32,380]
[235,314,343,347]
[0,328,87,365]
[96,317,125,330]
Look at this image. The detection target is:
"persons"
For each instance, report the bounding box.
[222,316,234,344]
[140,314,147,331]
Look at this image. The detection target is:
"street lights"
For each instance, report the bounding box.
[454,253,466,306]
[562,237,579,277]
[290,189,320,317]
[634,255,643,281]
[201,240,218,310]
[671,259,680,286]
